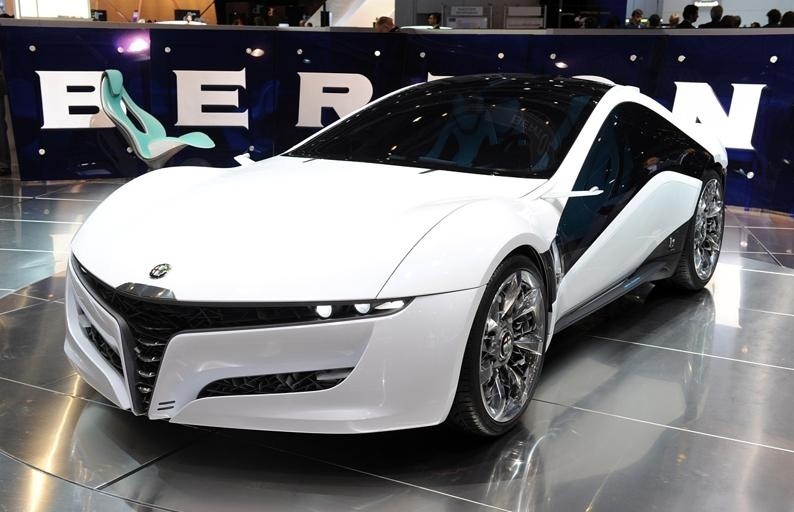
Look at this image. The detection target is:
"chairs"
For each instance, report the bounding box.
[100,69,216,171]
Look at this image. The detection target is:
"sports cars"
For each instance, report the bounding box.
[74,286,727,510]
[53,69,736,448]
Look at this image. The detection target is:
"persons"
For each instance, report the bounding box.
[375,12,442,33]
[261,5,279,26]
[626,4,794,29]
